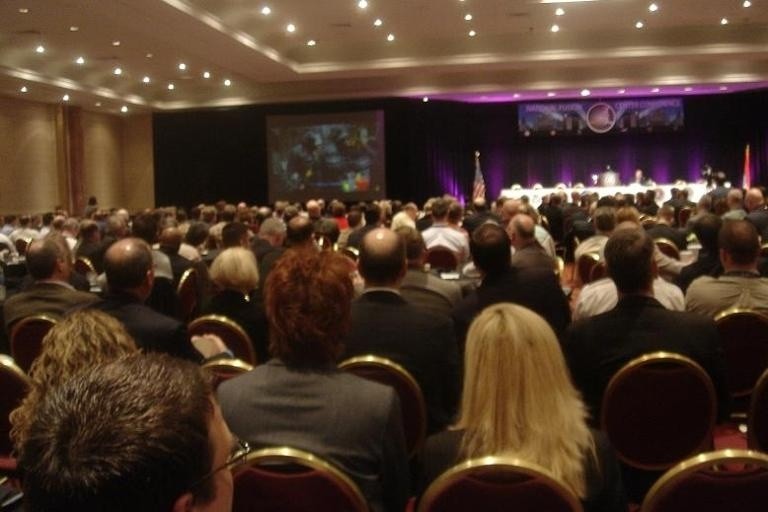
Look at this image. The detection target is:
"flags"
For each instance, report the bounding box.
[468,151,489,206]
[740,145,751,189]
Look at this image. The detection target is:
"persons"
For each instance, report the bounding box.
[4,190,768,449]
[632,168,645,184]
[217,243,407,512]
[416,299,628,510]
[7,348,254,511]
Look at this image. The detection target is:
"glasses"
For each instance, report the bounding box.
[193,431,252,495]
